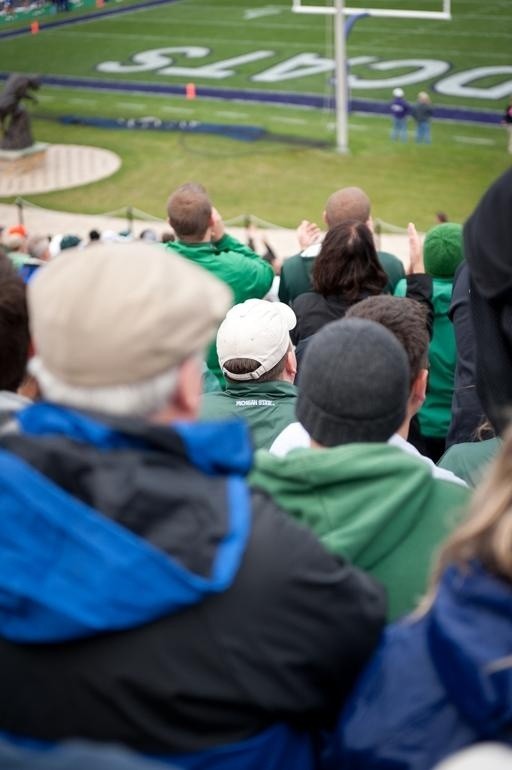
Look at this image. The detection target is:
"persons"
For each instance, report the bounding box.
[1,163,511,482]
[411,91,431,146]
[247,319,474,620]
[388,88,413,144]
[0,243,387,770]
[337,425,511,770]
[501,105,512,155]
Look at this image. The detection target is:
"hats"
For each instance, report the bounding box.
[216,298,297,381]
[25,239,234,388]
[423,223,463,277]
[295,318,411,447]
